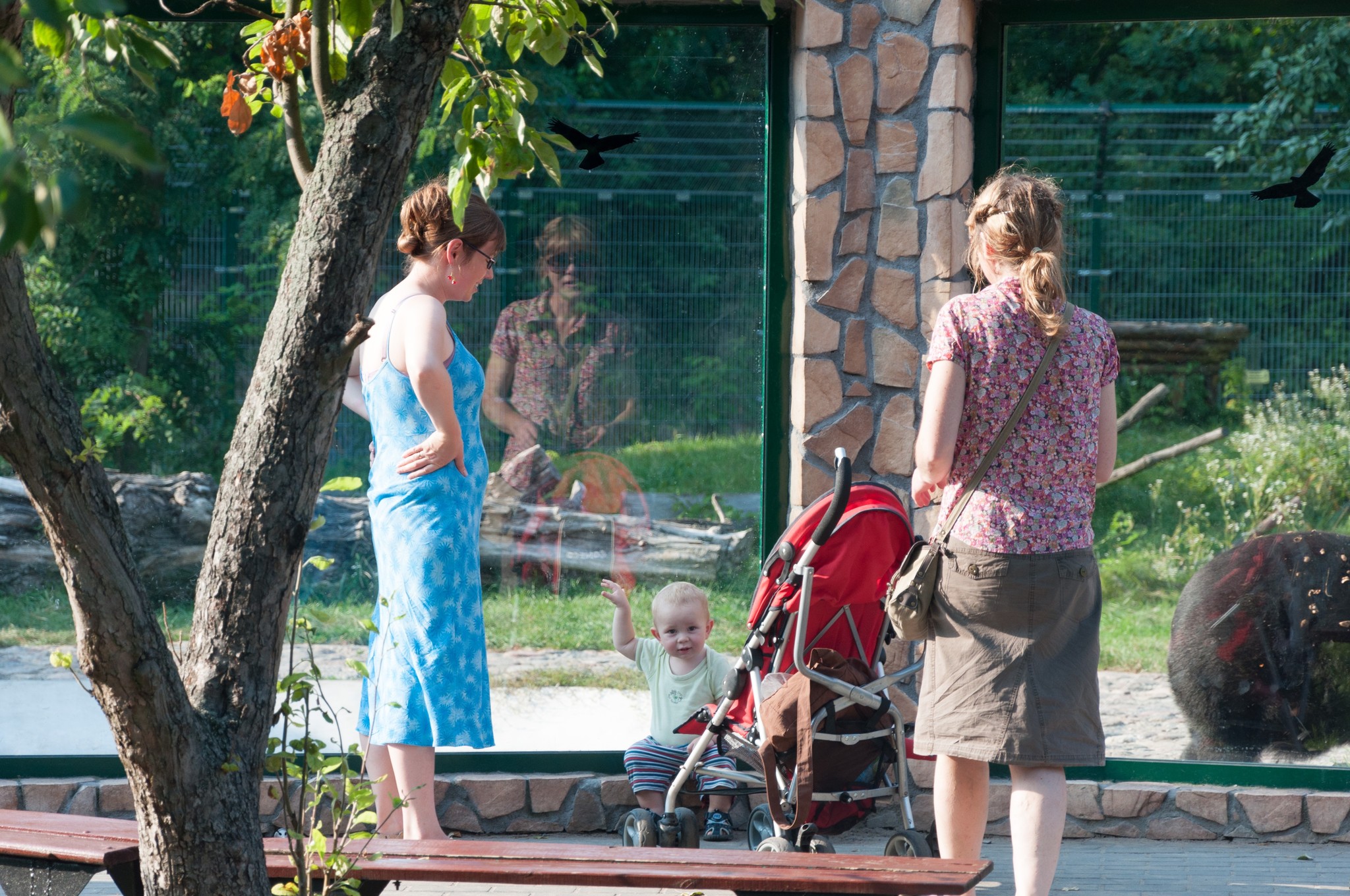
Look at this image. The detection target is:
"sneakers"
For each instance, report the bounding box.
[702,808,735,842]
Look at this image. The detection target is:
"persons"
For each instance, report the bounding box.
[599,575,744,842]
[905,173,1122,896]
[340,175,504,842]
[476,203,648,584]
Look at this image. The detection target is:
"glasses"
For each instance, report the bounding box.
[544,252,598,273]
[457,236,499,271]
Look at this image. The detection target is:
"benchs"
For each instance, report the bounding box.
[264,830,992,895]
[3,802,140,886]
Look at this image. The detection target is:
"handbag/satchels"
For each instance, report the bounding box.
[777,764,878,835]
[540,447,654,602]
[754,641,898,835]
[879,534,946,646]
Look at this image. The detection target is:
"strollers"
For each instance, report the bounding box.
[614,448,938,860]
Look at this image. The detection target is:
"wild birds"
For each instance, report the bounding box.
[1249,141,1337,208]
[546,116,642,170]
